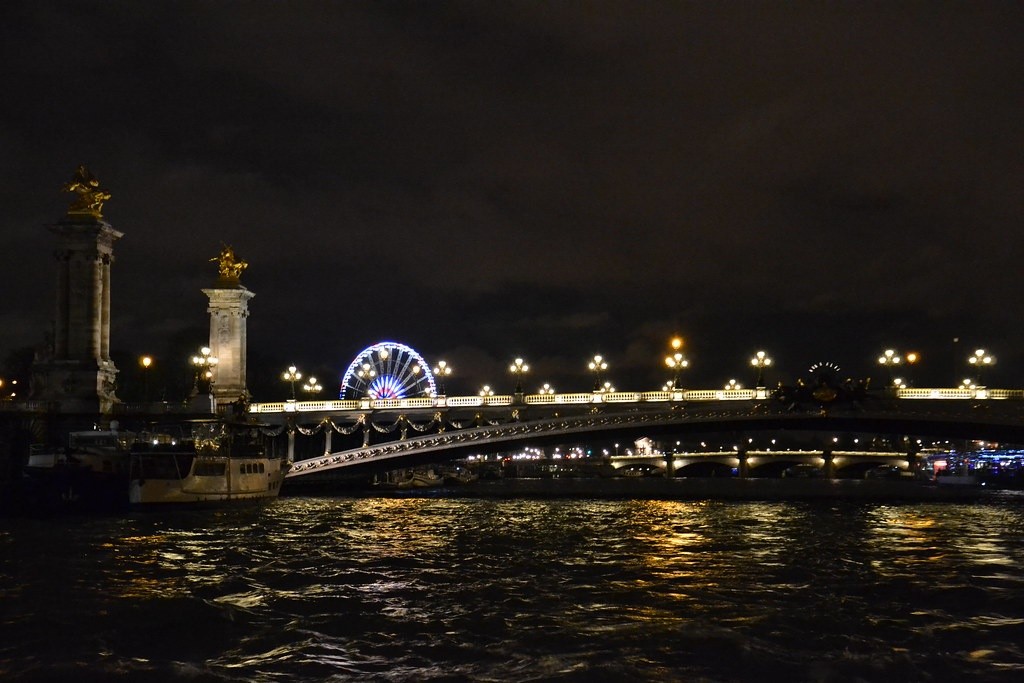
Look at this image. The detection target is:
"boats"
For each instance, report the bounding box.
[123,419,292,504]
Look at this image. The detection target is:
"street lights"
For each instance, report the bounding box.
[282,366,303,401]
[302,377,322,400]
[585,353,611,390]
[904,351,922,385]
[139,356,153,401]
[507,358,532,394]
[668,340,691,390]
[875,349,906,387]
[431,357,453,396]
[967,349,998,387]
[746,348,775,388]
[11,379,17,401]
